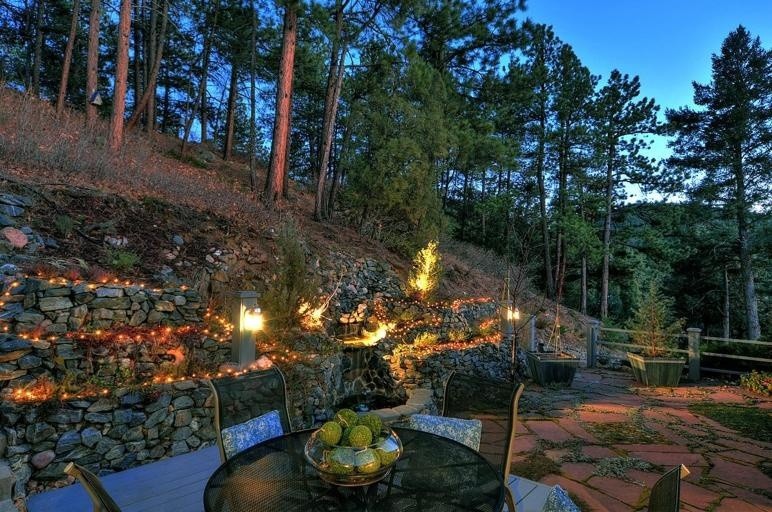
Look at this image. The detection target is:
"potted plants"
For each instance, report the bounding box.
[626,278,686,387]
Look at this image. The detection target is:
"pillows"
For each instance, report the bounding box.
[221,408,285,461]
[542,484,581,512]
[410,414,482,453]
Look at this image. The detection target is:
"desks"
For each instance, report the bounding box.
[204,423,505,512]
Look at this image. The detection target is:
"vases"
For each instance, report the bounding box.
[524,351,582,387]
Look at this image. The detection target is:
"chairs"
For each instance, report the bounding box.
[443,370,525,510]
[64,461,124,511]
[207,364,294,465]
[648,463,690,511]
